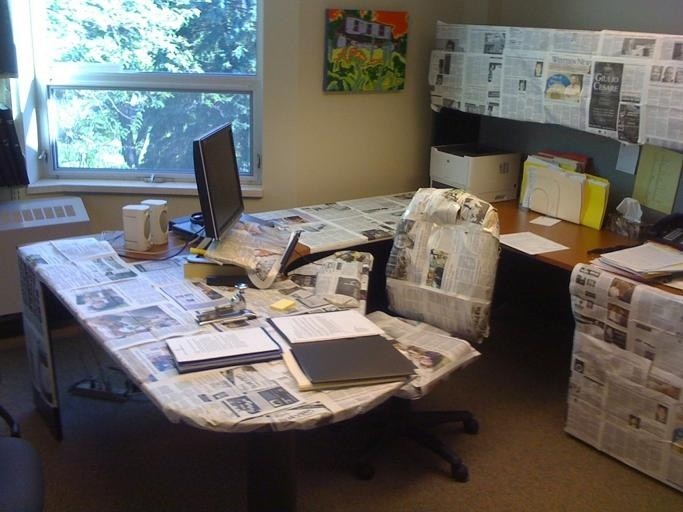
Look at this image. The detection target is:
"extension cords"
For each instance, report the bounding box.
[75,380,129,401]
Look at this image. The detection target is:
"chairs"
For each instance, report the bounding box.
[341,185,503,484]
[0,406,43,512]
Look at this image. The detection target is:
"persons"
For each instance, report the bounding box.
[392,339,438,368]
[563,73,582,103]
[650,65,682,85]
[518,80,527,92]
[533,61,542,78]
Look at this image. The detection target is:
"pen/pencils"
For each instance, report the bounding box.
[587,244,640,254]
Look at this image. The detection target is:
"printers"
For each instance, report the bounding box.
[430,141,521,203]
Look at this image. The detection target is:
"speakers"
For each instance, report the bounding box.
[122,204,152,251]
[141,199,169,245]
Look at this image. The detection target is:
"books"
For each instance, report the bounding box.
[517,154,610,233]
[524,168,587,224]
[289,334,419,384]
[280,350,409,393]
[164,326,282,374]
[260,308,384,346]
[536,149,589,173]
[588,257,640,280]
[598,243,682,280]
[531,154,584,172]
[187,306,256,327]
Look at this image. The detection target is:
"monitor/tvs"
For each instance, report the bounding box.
[187,121,245,264]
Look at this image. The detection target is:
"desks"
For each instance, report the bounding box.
[246,191,683,331]
[15,228,420,510]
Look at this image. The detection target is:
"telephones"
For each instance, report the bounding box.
[646,212,682,251]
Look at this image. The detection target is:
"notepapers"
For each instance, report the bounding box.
[269,299,296,311]
